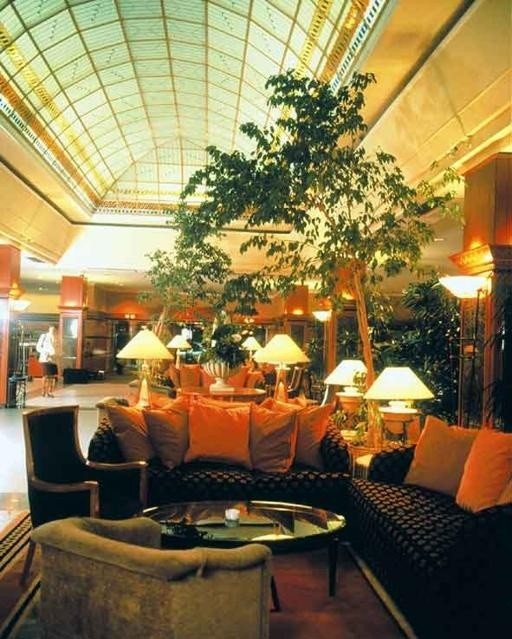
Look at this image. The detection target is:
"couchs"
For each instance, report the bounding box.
[343,436,511,639]
[145,371,263,409]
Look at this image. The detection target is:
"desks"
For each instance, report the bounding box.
[139,499,348,612]
[173,383,266,403]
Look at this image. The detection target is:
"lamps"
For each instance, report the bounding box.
[114,328,175,407]
[324,360,368,393]
[251,333,311,402]
[167,334,192,367]
[239,336,262,362]
[363,367,436,435]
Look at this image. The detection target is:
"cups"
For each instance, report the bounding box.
[224,521,240,528]
[224,508,240,519]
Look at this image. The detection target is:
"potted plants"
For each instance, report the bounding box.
[182,324,265,392]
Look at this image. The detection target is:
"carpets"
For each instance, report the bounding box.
[1,502,416,639]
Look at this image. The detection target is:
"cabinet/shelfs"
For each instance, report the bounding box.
[59,314,112,382]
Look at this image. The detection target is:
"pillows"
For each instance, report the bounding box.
[455,427,511,513]
[101,395,334,473]
[405,415,477,509]
[169,365,251,388]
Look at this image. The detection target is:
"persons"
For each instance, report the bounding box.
[36,321,59,398]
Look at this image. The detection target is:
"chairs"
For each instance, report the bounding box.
[31,517,274,638]
[22,404,146,581]
